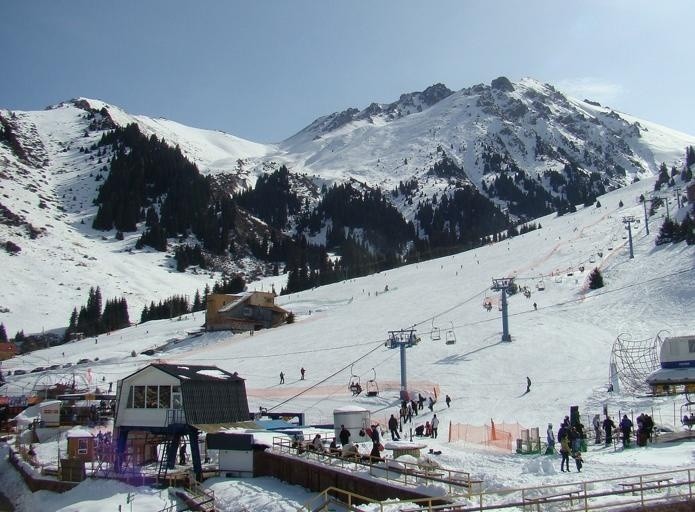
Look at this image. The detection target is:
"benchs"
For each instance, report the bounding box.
[286,434,484,499]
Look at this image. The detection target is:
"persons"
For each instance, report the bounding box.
[446,395,451,407]
[279,372,285,384]
[180,444,186,465]
[350,382,355,388]
[528,377,531,392]
[100,399,116,411]
[547,413,654,471]
[300,368,305,380]
[356,382,362,391]
[312,393,439,463]
